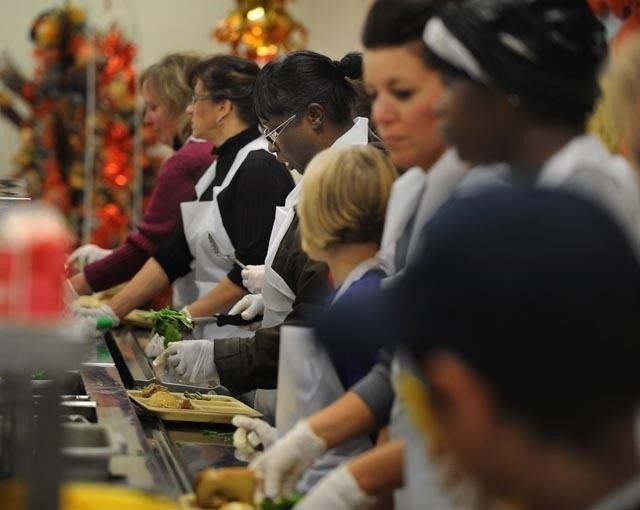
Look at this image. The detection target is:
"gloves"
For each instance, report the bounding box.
[241,264,265,294]
[232,415,278,463]
[152,340,220,382]
[248,418,326,505]
[67,243,113,269]
[228,294,264,331]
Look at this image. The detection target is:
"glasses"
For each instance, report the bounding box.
[191,93,214,105]
[263,114,296,146]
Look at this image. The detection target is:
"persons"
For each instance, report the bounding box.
[360,0,508,510]
[70,54,298,359]
[153,46,391,428]
[224,264,274,332]
[61,50,220,314]
[322,183,638,509]
[240,0,638,509]
[597,34,639,167]
[229,138,392,463]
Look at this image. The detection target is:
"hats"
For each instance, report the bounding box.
[421,1,608,133]
